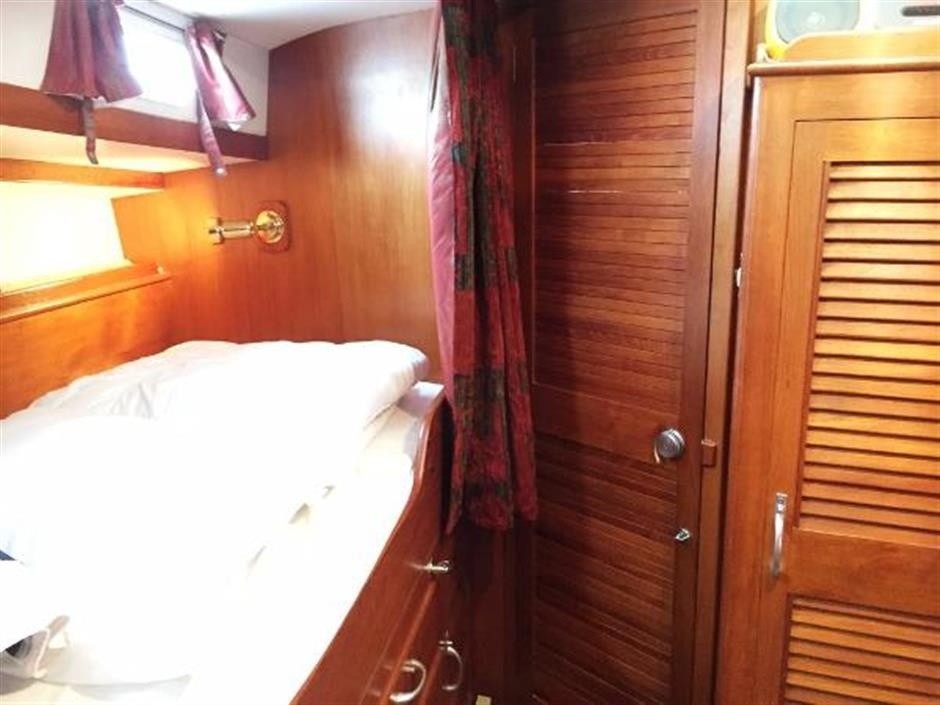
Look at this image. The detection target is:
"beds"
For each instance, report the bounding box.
[0,340,468,705]
